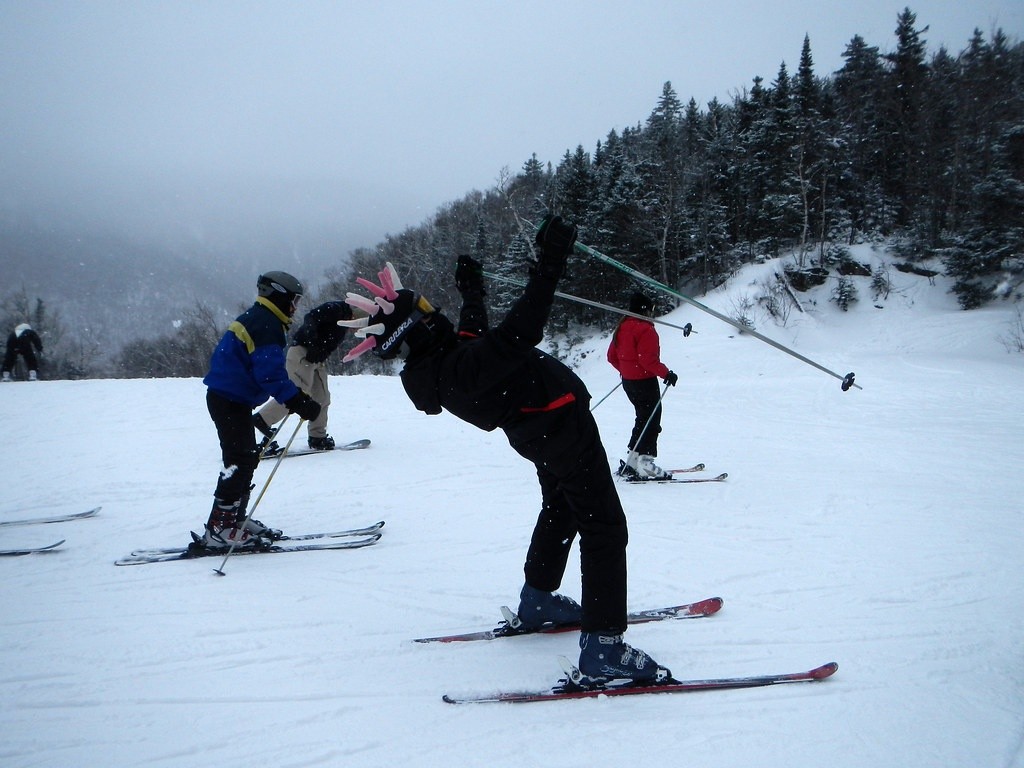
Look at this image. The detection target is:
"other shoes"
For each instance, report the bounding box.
[253,413,273,439]
[2,371,16,382]
[308,435,337,450]
[29,369,37,381]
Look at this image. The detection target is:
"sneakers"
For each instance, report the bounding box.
[578,631,673,681]
[518,587,583,630]
[635,454,673,481]
[624,451,640,476]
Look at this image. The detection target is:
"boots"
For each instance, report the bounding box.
[236,494,284,540]
[204,498,273,549]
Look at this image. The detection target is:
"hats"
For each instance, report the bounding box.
[352,308,369,319]
[337,261,437,363]
[256,271,304,317]
[629,291,656,315]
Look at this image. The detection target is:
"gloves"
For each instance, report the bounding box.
[536,215,580,260]
[284,387,321,422]
[455,255,488,298]
[663,370,678,386]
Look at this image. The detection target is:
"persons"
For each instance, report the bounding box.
[337,216,657,679]
[607,293,678,477]
[203,271,321,543]
[252,300,369,451]
[0,323,43,382]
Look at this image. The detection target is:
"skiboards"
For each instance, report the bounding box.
[0,507,101,554]
[115,521,386,569]
[415,598,839,706]
[258,437,370,460]
[615,464,728,484]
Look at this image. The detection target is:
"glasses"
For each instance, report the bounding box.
[416,291,435,316]
[289,294,304,309]
